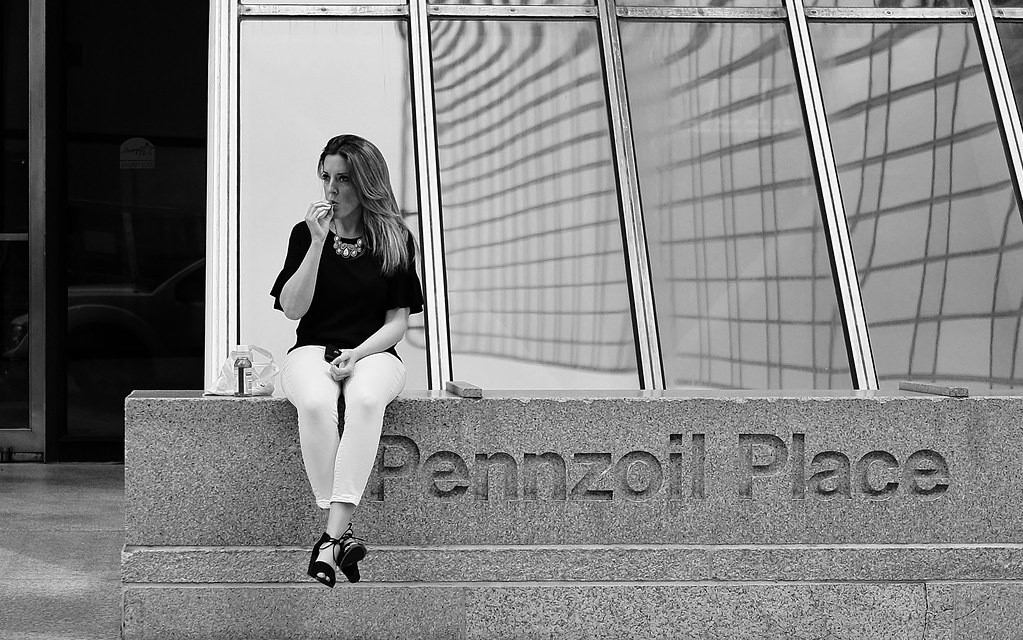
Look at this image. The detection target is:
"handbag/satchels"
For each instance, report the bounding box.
[203,343,279,395]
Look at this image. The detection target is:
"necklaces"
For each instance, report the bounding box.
[333,220,365,258]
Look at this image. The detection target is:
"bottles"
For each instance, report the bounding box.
[233,344,253,397]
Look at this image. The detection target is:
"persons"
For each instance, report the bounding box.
[269,135,424,588]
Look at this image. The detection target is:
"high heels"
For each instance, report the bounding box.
[307,522,366,588]
